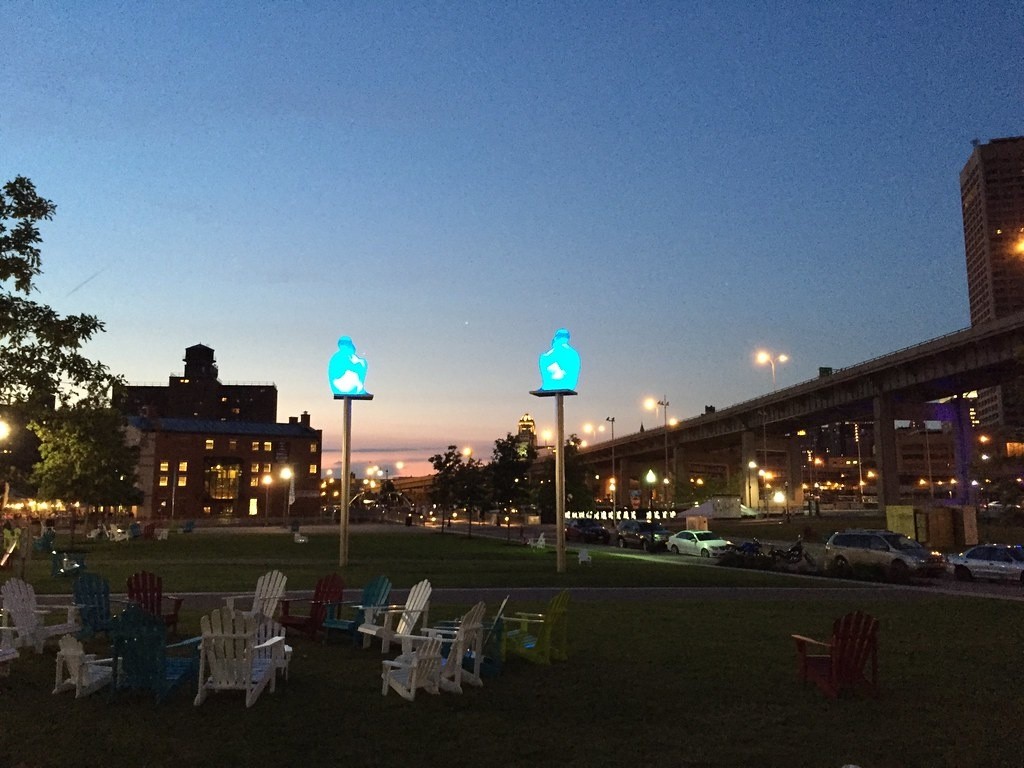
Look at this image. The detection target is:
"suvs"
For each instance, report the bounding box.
[822,528,946,584]
[613,518,674,554]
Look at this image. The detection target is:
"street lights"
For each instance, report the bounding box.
[525,327,582,573]
[748,460,757,509]
[643,467,659,555]
[280,468,291,528]
[325,335,376,567]
[262,474,272,527]
[655,393,671,528]
[754,351,788,391]
[606,416,616,528]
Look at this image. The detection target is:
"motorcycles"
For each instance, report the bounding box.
[768,535,816,568]
[720,534,773,571]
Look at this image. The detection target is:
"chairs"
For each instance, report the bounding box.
[357,579,432,654]
[34,521,58,553]
[52,634,122,699]
[51,550,86,578]
[109,603,198,710]
[791,610,882,703]
[381,586,578,701]
[290,519,310,544]
[87,519,194,542]
[321,573,393,644]
[125,571,184,633]
[193,606,294,708]
[0,577,86,679]
[221,571,287,620]
[280,572,346,642]
[73,574,140,640]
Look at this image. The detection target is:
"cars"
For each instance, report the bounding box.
[564,517,610,544]
[944,544,1024,584]
[664,530,734,559]
